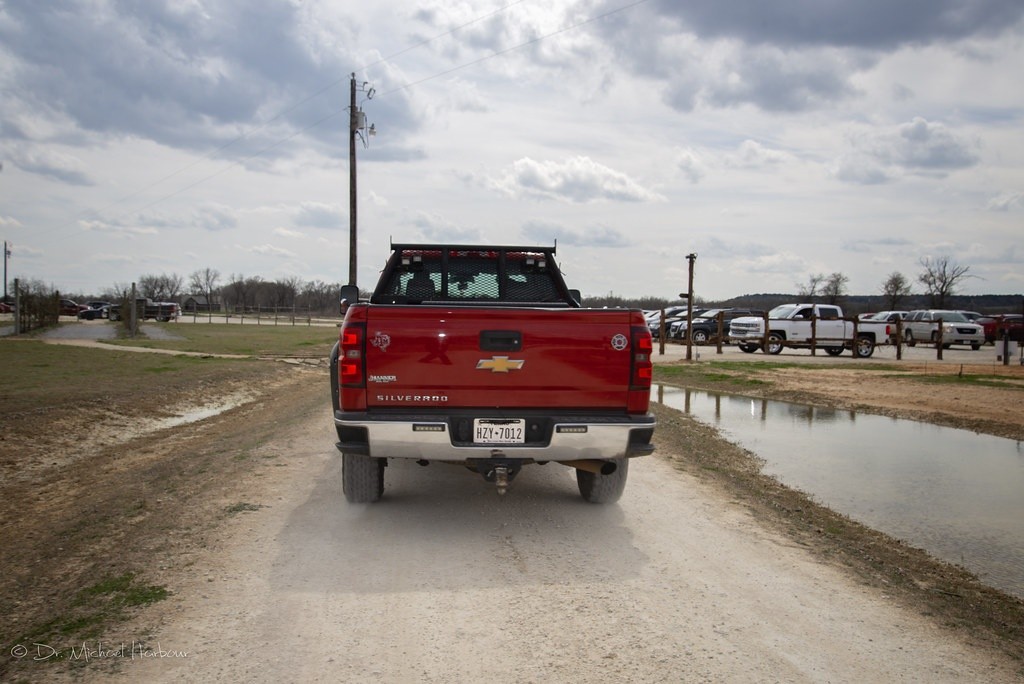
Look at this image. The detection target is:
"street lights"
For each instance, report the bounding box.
[349,122,376,285]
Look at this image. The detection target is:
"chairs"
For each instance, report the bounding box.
[406,270,436,299]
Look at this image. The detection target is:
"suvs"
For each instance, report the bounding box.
[639,305,753,347]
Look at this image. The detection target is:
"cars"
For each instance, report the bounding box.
[79,304,116,321]
[856,308,1024,350]
[59,299,93,316]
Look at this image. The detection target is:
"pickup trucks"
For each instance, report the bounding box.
[102,297,181,322]
[728,303,890,358]
[330,235,657,504]
